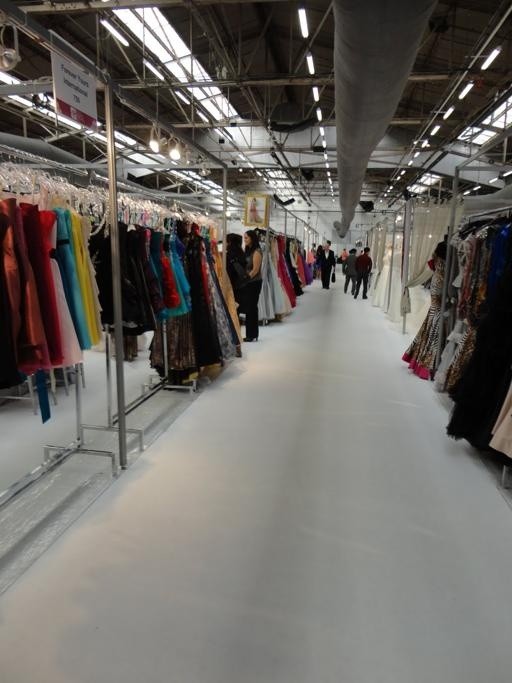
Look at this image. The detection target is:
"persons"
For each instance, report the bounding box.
[226,231,252,315]
[305,240,374,300]
[400,233,460,382]
[250,198,260,222]
[243,230,263,342]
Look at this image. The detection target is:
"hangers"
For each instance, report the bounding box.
[253,224,296,240]
[0,144,213,227]
[456,208,512,240]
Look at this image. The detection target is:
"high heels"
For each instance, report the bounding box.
[243,336,258,342]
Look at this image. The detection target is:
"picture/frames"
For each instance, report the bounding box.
[244,193,268,227]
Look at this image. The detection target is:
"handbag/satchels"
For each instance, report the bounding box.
[332,272,335,282]
[228,258,245,286]
